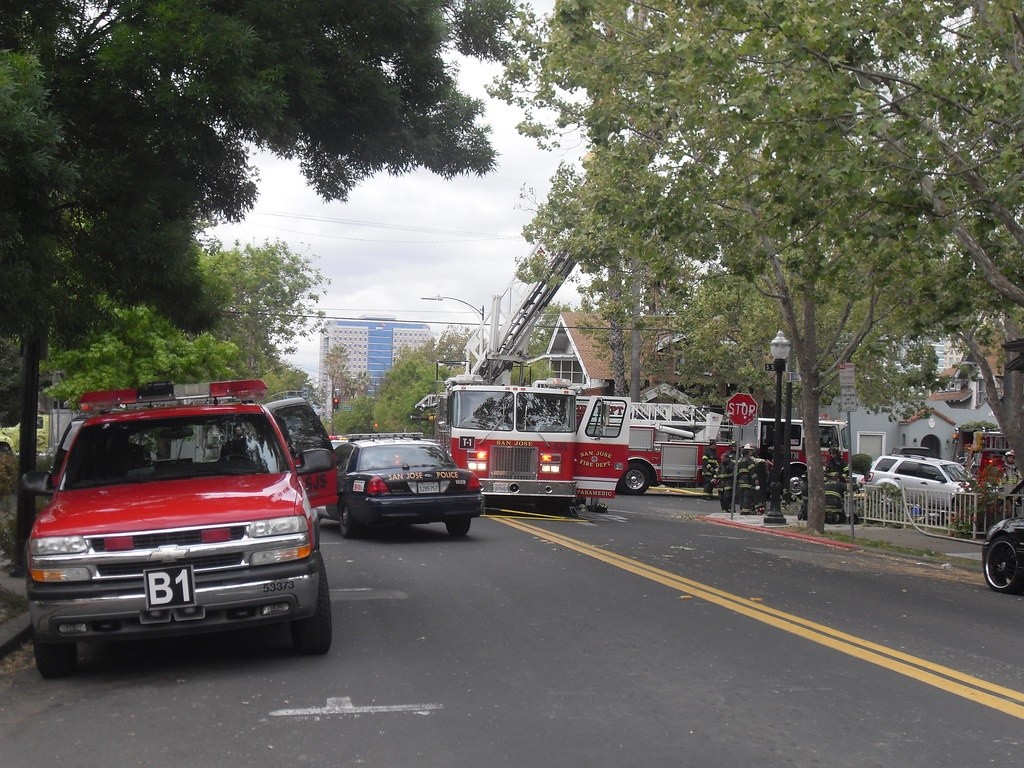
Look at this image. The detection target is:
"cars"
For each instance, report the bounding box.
[981,517,1024,594]
[314,430,486,540]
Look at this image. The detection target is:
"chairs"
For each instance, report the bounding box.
[103,444,145,477]
[220,438,258,468]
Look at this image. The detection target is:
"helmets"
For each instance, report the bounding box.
[708,439,716,444]
[828,446,839,453]
[744,444,754,449]
[729,441,737,446]
[1005,452,1014,457]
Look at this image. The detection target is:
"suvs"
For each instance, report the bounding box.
[16,376,340,679]
[851,451,993,526]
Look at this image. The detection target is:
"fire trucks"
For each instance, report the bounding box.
[965,429,1017,520]
[408,372,633,515]
[576,403,849,506]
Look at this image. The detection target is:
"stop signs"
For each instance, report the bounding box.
[725,392,759,426]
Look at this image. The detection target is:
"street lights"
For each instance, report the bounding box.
[421,294,484,357]
[762,330,791,523]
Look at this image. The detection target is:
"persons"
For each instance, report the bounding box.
[700,439,771,515]
[996,449,1022,517]
[798,444,851,523]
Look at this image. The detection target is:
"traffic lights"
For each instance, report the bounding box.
[333,397,340,409]
